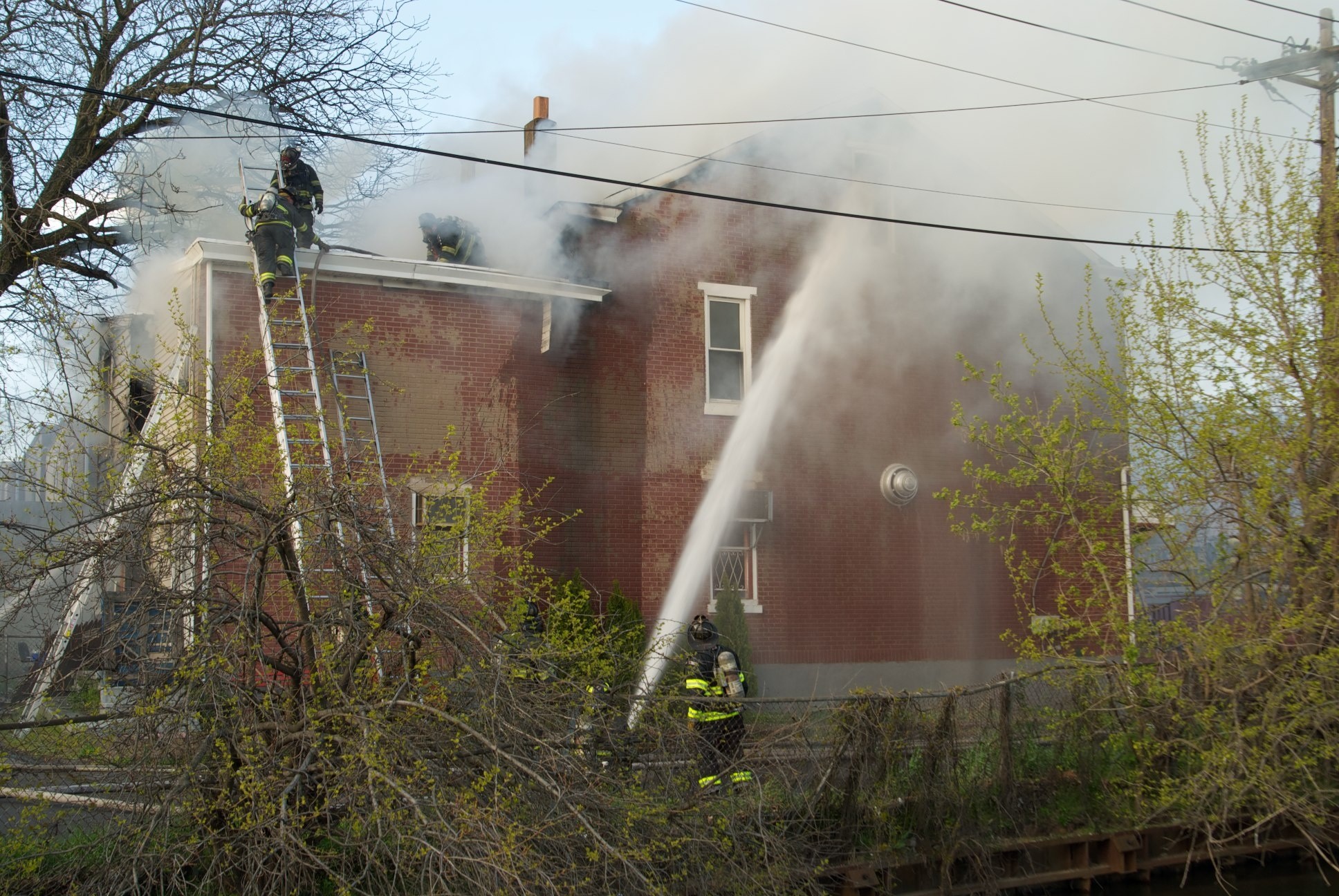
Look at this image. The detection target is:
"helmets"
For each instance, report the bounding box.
[418,213,442,229]
[687,614,719,651]
[277,188,297,205]
[281,146,301,169]
[514,601,543,633]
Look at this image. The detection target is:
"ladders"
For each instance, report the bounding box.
[12,324,199,740]
[326,346,416,684]
[237,154,348,646]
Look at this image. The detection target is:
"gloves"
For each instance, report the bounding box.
[315,238,331,252]
[238,196,251,212]
[314,203,323,214]
[284,185,300,196]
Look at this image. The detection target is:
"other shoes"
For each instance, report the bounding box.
[264,282,273,304]
[280,264,294,276]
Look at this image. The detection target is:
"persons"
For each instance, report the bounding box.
[270,146,324,250]
[238,186,331,304]
[417,213,486,266]
[684,615,749,823]
